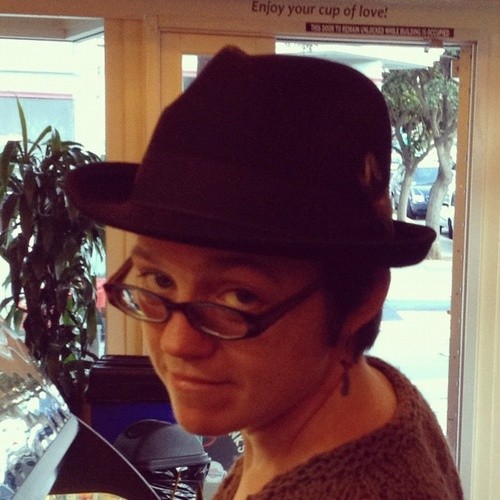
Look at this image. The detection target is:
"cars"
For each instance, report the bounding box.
[389,142,457,240]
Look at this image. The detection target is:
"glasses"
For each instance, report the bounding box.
[103,256,335,341]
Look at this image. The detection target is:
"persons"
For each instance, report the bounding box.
[101,83,465,500]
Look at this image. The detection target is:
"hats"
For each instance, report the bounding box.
[65,45,436,267]
